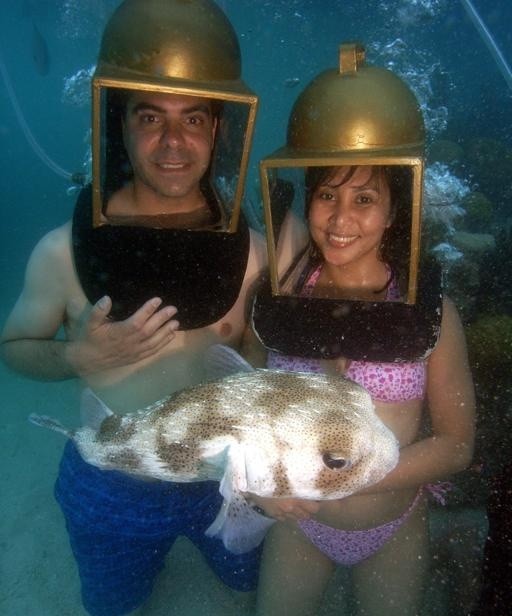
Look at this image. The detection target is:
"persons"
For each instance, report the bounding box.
[0,90,268,613]
[239,165,476,614]
